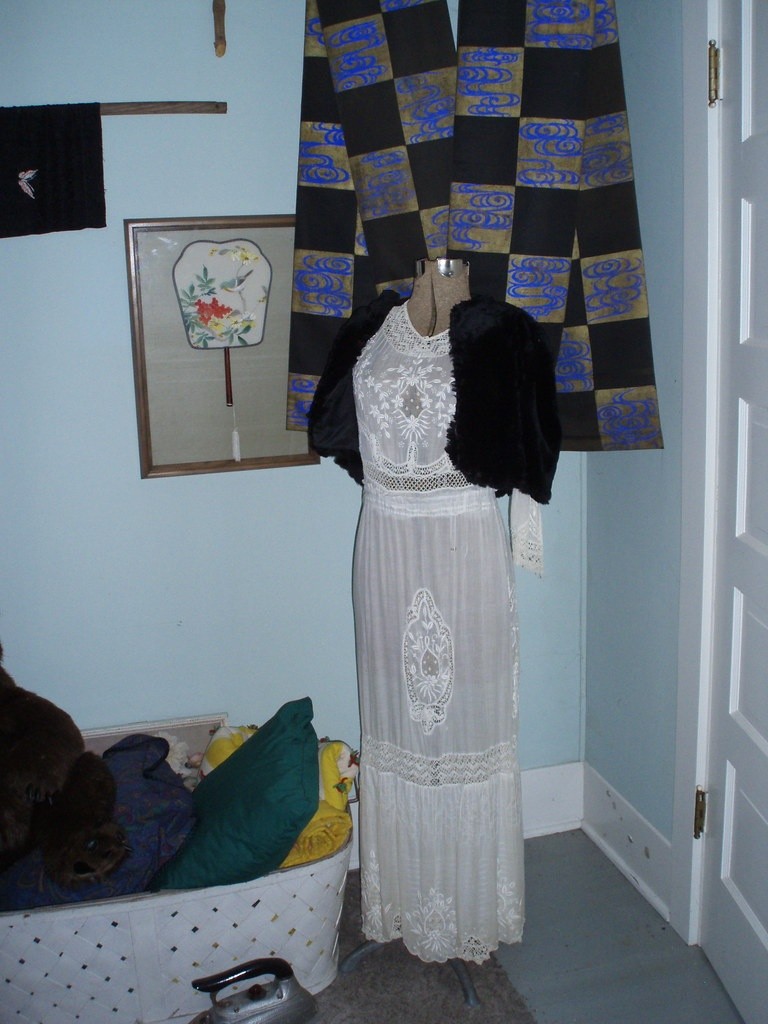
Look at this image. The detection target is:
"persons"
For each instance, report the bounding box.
[307,254,562,965]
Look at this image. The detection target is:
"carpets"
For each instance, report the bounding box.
[303,865,538,1024]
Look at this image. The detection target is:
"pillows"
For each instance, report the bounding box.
[155,697,319,892]
[12,734,196,911]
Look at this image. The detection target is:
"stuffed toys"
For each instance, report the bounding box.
[0,662,136,885]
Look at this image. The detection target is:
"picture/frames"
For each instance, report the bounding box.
[122,216,321,480]
